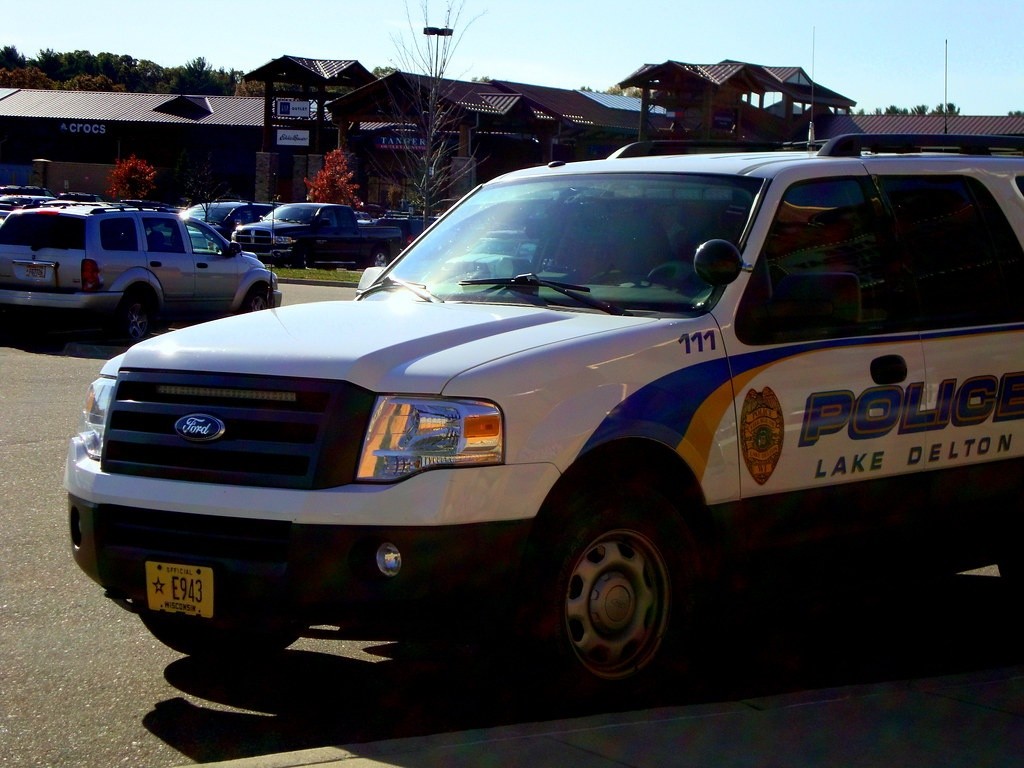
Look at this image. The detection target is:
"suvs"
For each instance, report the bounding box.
[0,207,279,340]
[66,134,1024,711]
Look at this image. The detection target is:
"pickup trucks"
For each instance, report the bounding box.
[232,202,401,269]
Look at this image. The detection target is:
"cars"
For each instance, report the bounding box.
[0,184,272,242]
